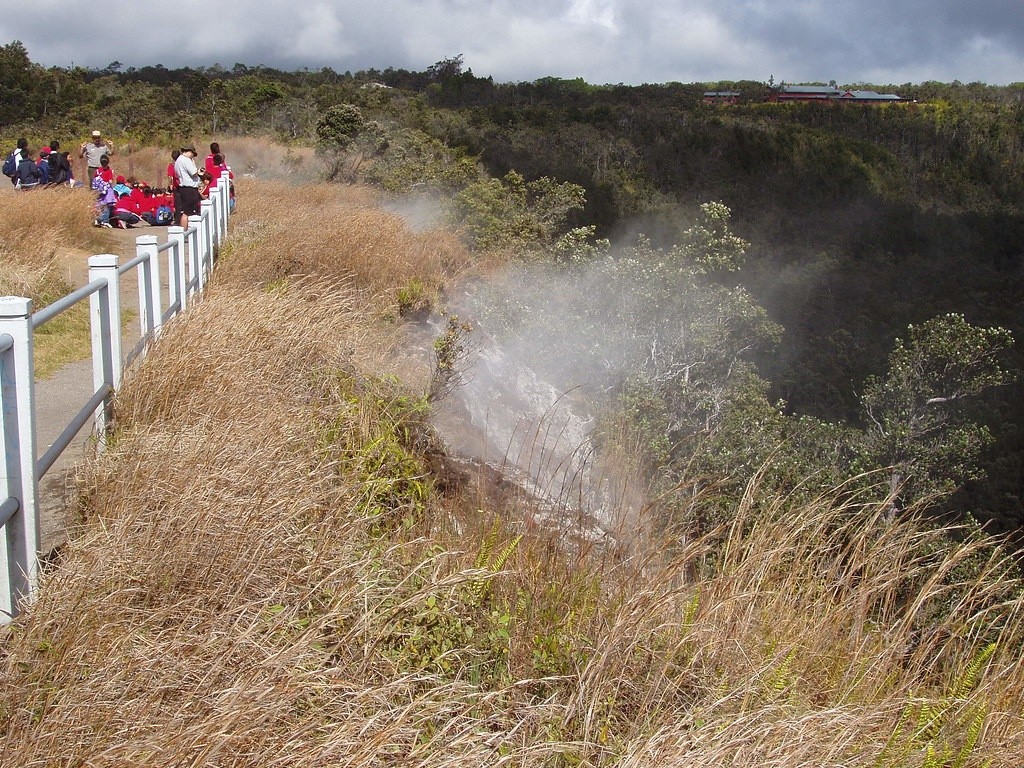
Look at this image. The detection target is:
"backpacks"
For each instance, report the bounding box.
[91,169,109,194]
[2,148,20,178]
[156,204,173,225]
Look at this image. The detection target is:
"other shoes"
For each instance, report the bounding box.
[94,219,102,226]
[118,220,127,229]
[101,222,113,229]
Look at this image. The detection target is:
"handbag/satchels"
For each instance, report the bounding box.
[12,166,21,190]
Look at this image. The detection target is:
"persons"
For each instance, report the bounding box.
[197,142,235,210]
[176,148,200,243]
[9,138,73,190]
[110,175,176,230]
[166,150,183,226]
[79,130,114,189]
[93,154,114,228]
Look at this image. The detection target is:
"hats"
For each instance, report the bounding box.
[141,181,147,187]
[116,176,127,184]
[181,145,197,157]
[92,130,101,136]
[41,146,51,154]
